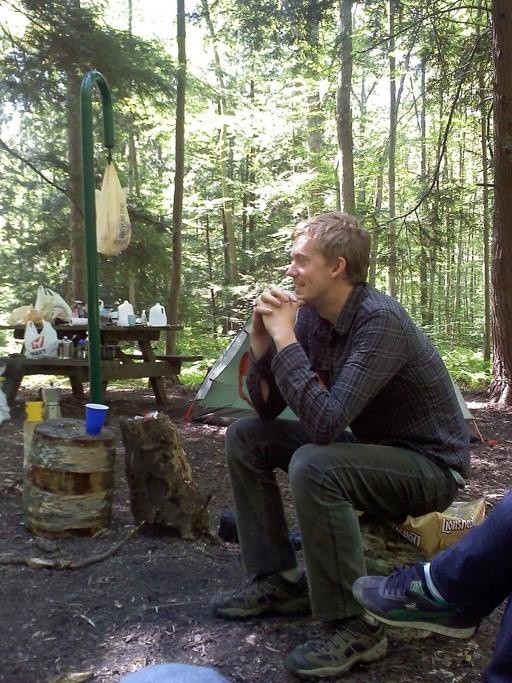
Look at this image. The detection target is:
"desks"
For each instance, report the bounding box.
[0,326,182,406]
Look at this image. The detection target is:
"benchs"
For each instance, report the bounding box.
[1,357,103,409]
[121,355,203,406]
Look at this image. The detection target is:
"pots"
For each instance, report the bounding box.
[41,381,64,407]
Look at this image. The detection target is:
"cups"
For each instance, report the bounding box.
[24,400,46,421]
[82,401,111,434]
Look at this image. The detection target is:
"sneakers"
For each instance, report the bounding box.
[283,616,388,678]
[210,568,311,620]
[353,562,478,639]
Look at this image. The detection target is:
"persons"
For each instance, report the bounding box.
[210,209,472,678]
[351,487,511,683]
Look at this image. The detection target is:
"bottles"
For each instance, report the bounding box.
[57,335,121,359]
[73,299,147,327]
